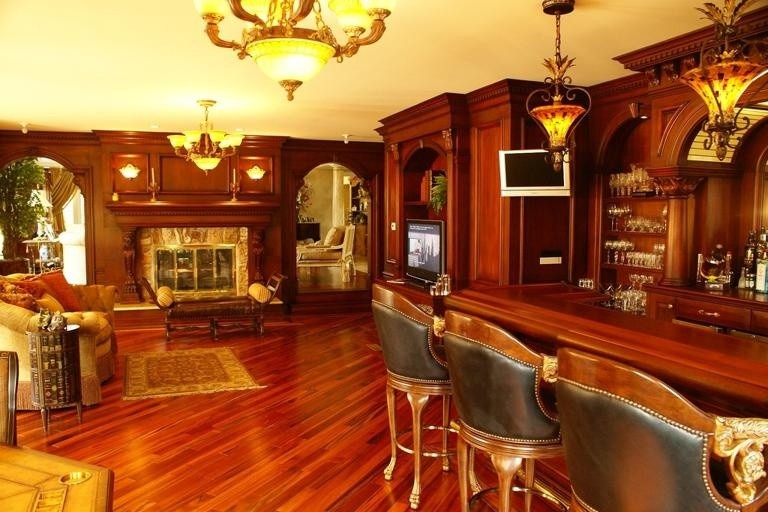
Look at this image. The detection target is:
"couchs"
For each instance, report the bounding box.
[0,273,121,414]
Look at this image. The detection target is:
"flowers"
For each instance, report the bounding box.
[296,178,311,206]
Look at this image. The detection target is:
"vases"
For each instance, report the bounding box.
[296,207,300,222]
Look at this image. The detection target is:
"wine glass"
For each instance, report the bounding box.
[607,168,651,197]
[606,202,632,232]
[602,239,665,270]
[658,201,667,230]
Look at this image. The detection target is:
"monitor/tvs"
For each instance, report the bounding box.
[405,219,446,289]
[498,149,571,197]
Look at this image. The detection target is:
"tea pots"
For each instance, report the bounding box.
[48,310,68,332]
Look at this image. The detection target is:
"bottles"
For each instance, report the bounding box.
[738,224,768,294]
[701,243,726,278]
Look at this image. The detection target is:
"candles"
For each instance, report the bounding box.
[151,168,156,186]
[232,168,236,184]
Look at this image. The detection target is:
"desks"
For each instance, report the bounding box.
[0,445,115,512]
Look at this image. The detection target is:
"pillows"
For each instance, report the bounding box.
[0,269,80,317]
[156,286,175,307]
[248,282,271,304]
[322,224,345,247]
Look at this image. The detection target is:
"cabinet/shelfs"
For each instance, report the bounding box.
[638,280,767,337]
[350,186,369,224]
[600,192,668,276]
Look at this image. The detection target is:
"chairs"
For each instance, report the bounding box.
[371,280,767,511]
[0,351,18,447]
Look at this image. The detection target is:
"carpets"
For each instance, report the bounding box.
[121,346,255,402]
[296,266,369,295]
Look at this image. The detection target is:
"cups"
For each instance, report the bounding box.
[605,274,652,313]
[627,214,663,232]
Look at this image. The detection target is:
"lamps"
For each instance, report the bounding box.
[519,0,595,171]
[680,1,768,164]
[166,1,390,177]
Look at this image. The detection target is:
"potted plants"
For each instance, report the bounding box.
[0,158,47,273]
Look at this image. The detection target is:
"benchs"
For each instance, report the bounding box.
[140,270,289,342]
